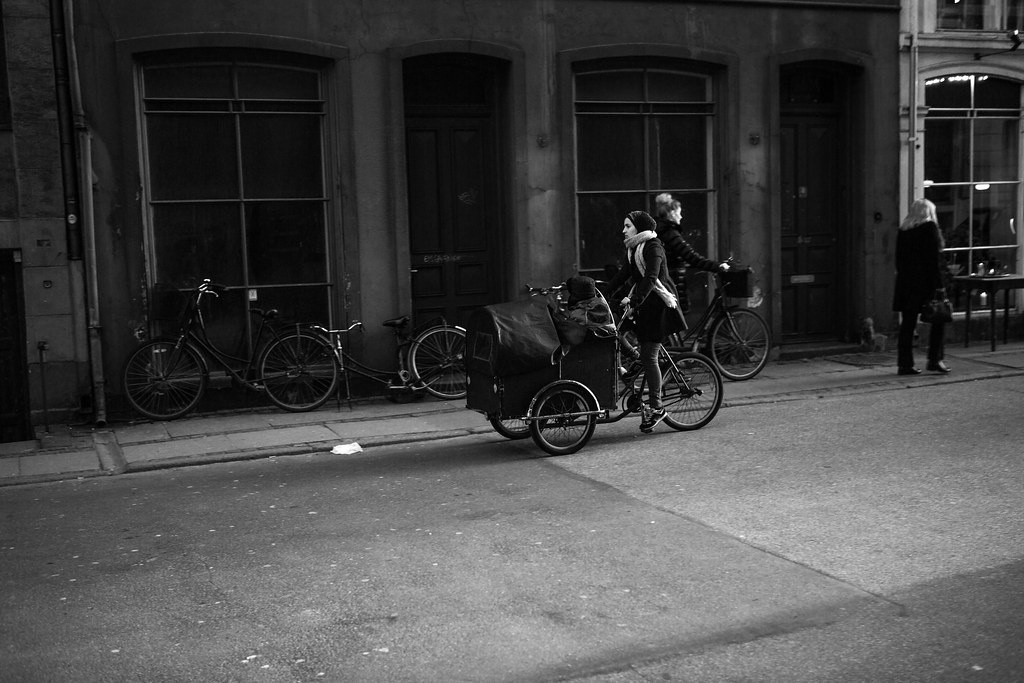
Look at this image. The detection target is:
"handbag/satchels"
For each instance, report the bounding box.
[919,289,954,324]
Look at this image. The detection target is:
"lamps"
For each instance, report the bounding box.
[973,30,1023,60]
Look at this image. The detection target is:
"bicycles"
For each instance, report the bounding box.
[121,277,343,421]
[616,256,773,395]
[306,314,468,413]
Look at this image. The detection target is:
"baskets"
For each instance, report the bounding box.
[717,266,755,298]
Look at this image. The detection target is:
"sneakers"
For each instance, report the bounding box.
[639,407,667,430]
[622,360,645,380]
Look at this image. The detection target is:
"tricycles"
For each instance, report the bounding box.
[486,276,723,455]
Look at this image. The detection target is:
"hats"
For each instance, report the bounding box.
[566,276,595,309]
[627,210,656,233]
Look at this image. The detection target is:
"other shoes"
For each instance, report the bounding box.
[926,359,952,372]
[898,366,923,375]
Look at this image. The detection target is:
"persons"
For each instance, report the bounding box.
[654,193,729,366]
[891,200,951,375]
[563,275,610,333]
[603,210,688,430]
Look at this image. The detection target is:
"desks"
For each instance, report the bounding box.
[952,274,1024,353]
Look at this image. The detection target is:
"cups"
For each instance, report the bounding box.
[977,264,985,276]
